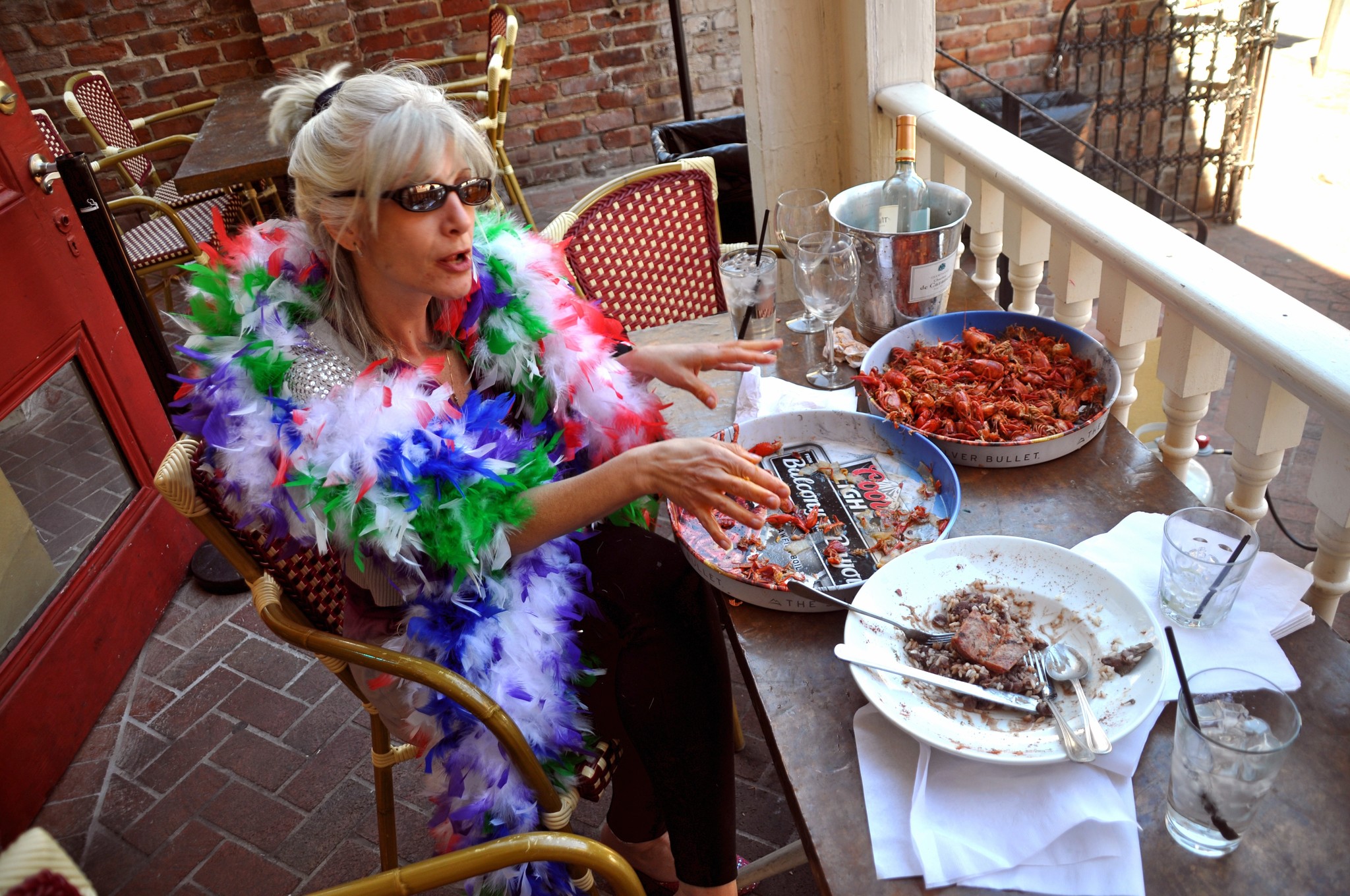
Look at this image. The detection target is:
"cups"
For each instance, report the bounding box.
[717,248,779,354]
[1158,507,1261,629]
[1164,667,1302,858]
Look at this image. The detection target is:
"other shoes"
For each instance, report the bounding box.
[638,853,760,893]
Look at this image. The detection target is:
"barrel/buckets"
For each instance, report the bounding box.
[828,180,973,344]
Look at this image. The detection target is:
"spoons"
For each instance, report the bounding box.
[1045,644,1113,755]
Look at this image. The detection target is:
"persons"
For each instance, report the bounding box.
[168,62,791,896]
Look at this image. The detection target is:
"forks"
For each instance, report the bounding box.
[1022,649,1096,763]
[786,579,956,646]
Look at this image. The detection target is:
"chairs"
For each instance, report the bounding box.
[445,33,539,237]
[394,2,519,205]
[0,825,649,896]
[151,429,748,896]
[64,69,288,224]
[31,109,253,334]
[538,155,727,334]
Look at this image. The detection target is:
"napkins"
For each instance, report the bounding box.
[855,511,1315,896]
[734,366,858,426]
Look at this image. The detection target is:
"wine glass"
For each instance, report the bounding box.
[792,231,858,389]
[774,188,835,333]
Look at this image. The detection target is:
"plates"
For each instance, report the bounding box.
[666,409,962,613]
[859,311,1123,468]
[843,536,1166,767]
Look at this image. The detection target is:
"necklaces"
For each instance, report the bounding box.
[385,346,460,407]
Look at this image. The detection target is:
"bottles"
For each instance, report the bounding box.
[878,116,931,234]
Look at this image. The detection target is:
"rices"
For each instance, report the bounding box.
[901,590,1141,723]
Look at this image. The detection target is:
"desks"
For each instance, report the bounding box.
[174,74,290,197]
[627,271,1350,896]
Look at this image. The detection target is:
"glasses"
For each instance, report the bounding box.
[331,178,492,212]
